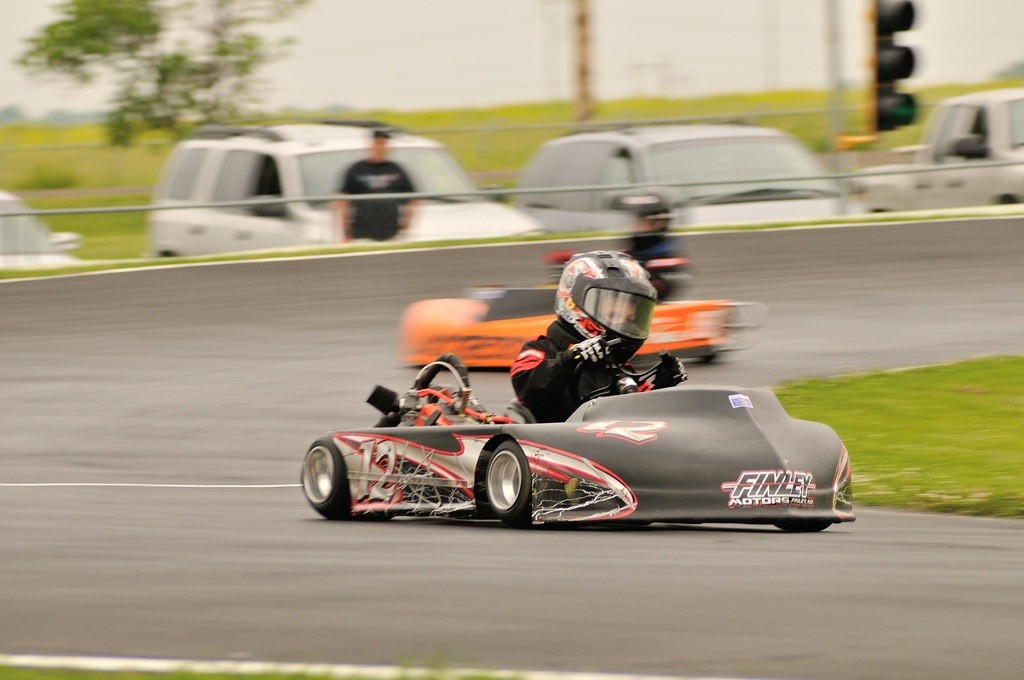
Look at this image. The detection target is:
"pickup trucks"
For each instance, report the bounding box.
[854,89,1024,214]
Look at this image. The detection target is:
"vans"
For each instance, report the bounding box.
[517,120,862,236]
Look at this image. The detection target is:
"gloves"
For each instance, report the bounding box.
[653,354,687,388]
[566,336,614,368]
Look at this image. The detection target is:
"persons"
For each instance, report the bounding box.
[505,251,687,424]
[624,195,681,300]
[339,131,416,242]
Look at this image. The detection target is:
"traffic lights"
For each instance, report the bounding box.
[866,2,917,137]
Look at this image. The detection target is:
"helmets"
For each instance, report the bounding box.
[555,249,657,364]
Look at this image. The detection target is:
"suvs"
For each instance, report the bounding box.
[151,123,543,258]
